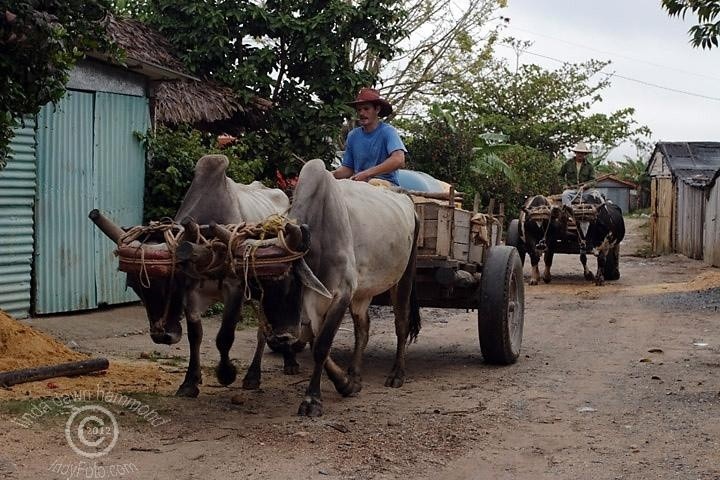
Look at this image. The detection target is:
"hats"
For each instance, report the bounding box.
[346,89,391,117]
[573,142,591,153]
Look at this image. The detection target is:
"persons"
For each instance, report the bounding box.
[558,140,593,190]
[322,85,408,190]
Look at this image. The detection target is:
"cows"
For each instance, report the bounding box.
[208,157,422,417]
[564,199,625,285]
[518,194,567,285]
[88,154,300,399]
